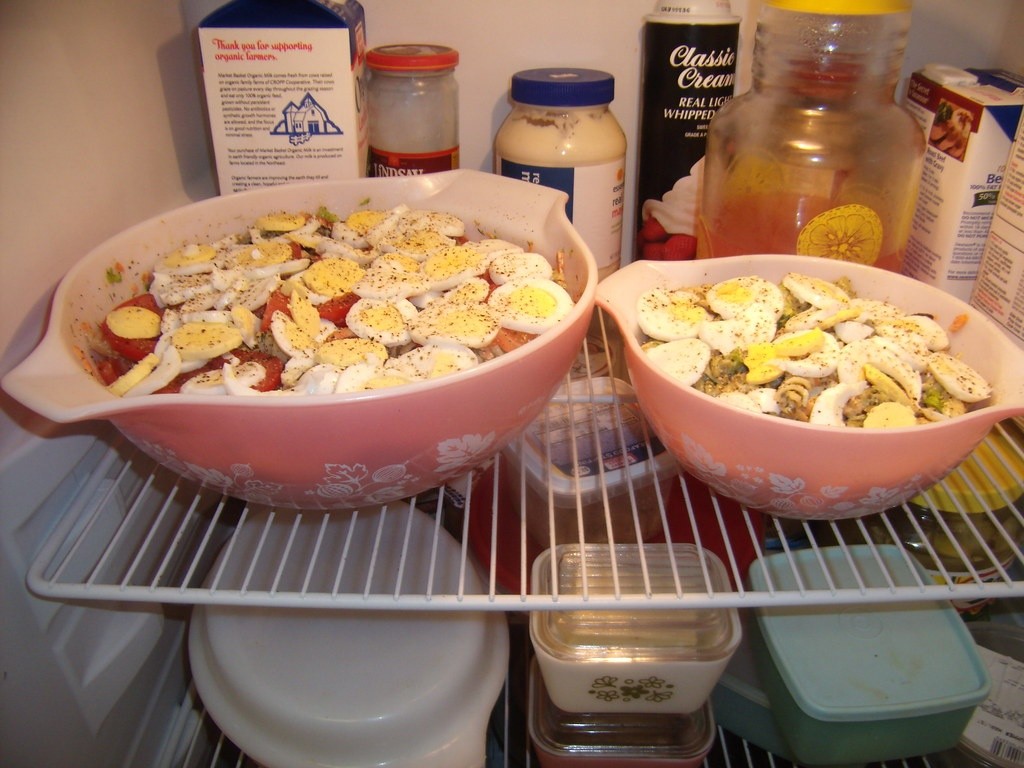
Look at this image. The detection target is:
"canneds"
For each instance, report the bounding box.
[494,67,625,283]
[363,42,460,177]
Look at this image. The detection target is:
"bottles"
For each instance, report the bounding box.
[698,0,926,274]
[633,2,741,252]
[495,69,626,275]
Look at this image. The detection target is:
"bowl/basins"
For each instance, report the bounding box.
[596,255,1024,520]
[0,169,596,511]
[180,534,991,768]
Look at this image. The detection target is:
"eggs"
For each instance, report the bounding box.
[102,211,574,398]
[635,269,991,430]
